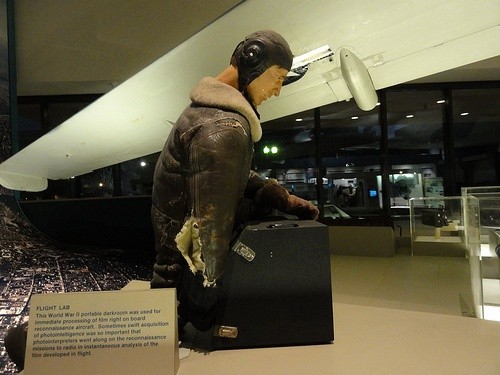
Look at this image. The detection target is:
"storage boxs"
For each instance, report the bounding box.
[181,220,335,352]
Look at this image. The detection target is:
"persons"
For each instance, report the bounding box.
[150,29,320,349]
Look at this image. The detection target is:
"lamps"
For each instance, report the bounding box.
[290,44,334,71]
